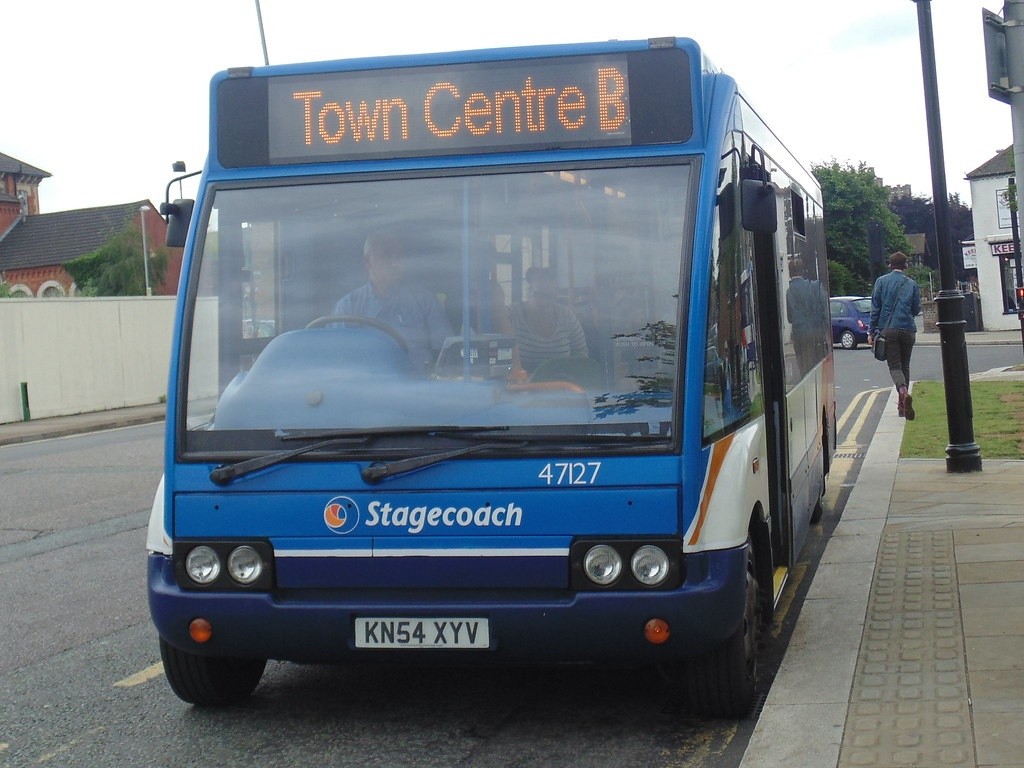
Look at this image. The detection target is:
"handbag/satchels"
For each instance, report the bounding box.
[871,335,887,361]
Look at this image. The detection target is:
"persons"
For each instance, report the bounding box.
[508,268,588,370]
[787,259,826,376]
[867,252,921,421]
[332,231,454,368]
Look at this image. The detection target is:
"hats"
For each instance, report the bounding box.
[789,259,804,275]
[888,251,909,269]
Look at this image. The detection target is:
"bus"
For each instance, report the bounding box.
[146,37,840,723]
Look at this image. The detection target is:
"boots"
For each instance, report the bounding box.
[898,385,915,421]
[898,399,904,417]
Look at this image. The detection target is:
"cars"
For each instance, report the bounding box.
[830,295,875,351]
